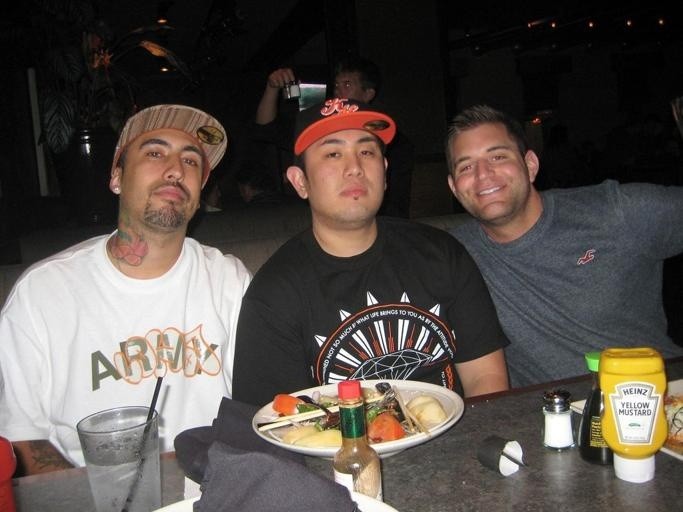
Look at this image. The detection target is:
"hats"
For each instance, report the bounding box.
[111,105,227,179]
[292,99,397,165]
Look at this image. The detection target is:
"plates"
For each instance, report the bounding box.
[568,379,683,463]
[252,378,464,455]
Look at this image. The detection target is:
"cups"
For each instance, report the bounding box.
[77,406,163,512]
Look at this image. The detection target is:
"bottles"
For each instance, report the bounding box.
[577,350,615,466]
[334,381,385,506]
[543,391,574,448]
[599,347,666,483]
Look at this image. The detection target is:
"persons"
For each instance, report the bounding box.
[251,57,414,219]
[231,97,511,408]
[0,102,254,475]
[237,162,300,206]
[196,175,227,216]
[442,101,683,390]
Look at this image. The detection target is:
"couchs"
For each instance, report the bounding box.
[0,214,466,312]
